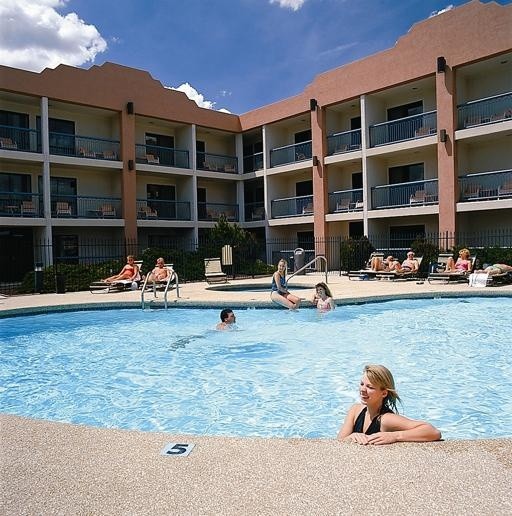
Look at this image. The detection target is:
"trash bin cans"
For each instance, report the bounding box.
[293,248,305,275]
[55,273,65,294]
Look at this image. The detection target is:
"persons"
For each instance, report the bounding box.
[385,251,419,273]
[474,261,512,274]
[336,365,441,444]
[100,255,139,282]
[148,257,170,284]
[371,256,393,271]
[444,248,472,273]
[315,283,336,310]
[215,309,235,330]
[270,260,301,309]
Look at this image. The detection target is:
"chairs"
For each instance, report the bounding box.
[139,264,173,291]
[252,144,364,221]
[349,253,512,286]
[90,261,143,294]
[0,136,158,218]
[204,258,227,284]
[409,111,512,206]
[204,160,236,222]
